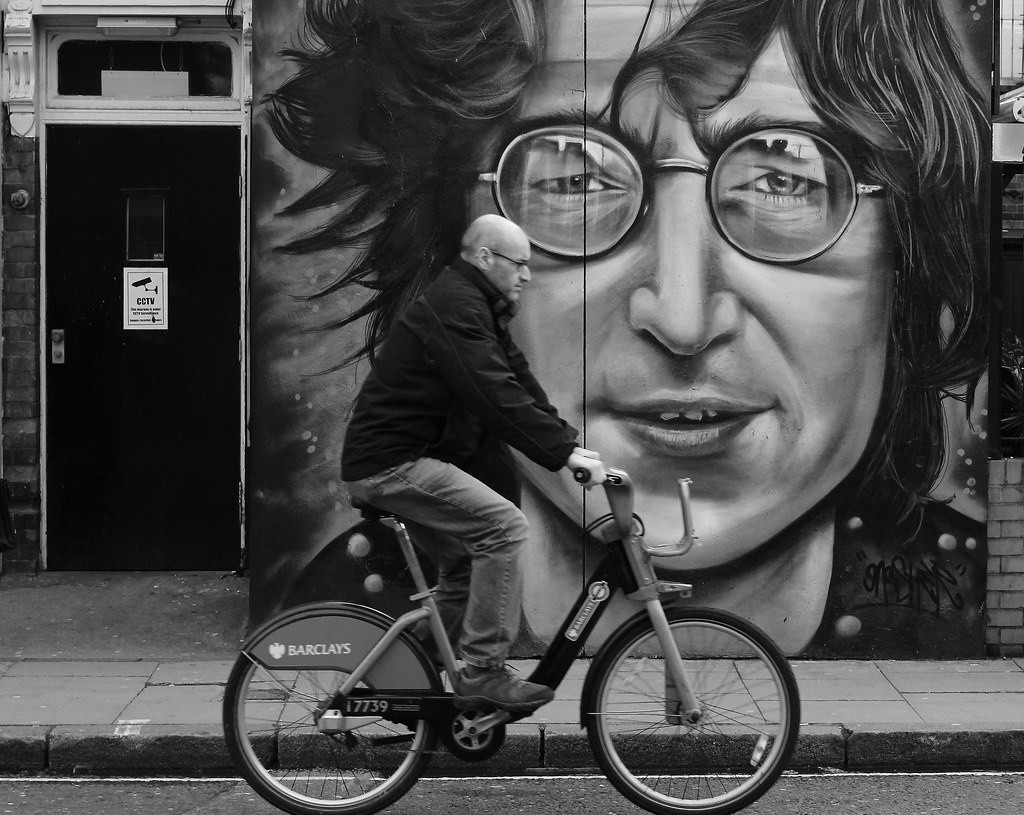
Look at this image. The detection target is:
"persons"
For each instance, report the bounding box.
[340,213,607,707]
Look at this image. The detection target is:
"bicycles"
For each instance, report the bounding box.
[222,466,801,815]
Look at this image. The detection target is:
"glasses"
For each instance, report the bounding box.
[490,250,531,271]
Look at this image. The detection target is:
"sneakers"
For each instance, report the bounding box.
[454,663,554,713]
